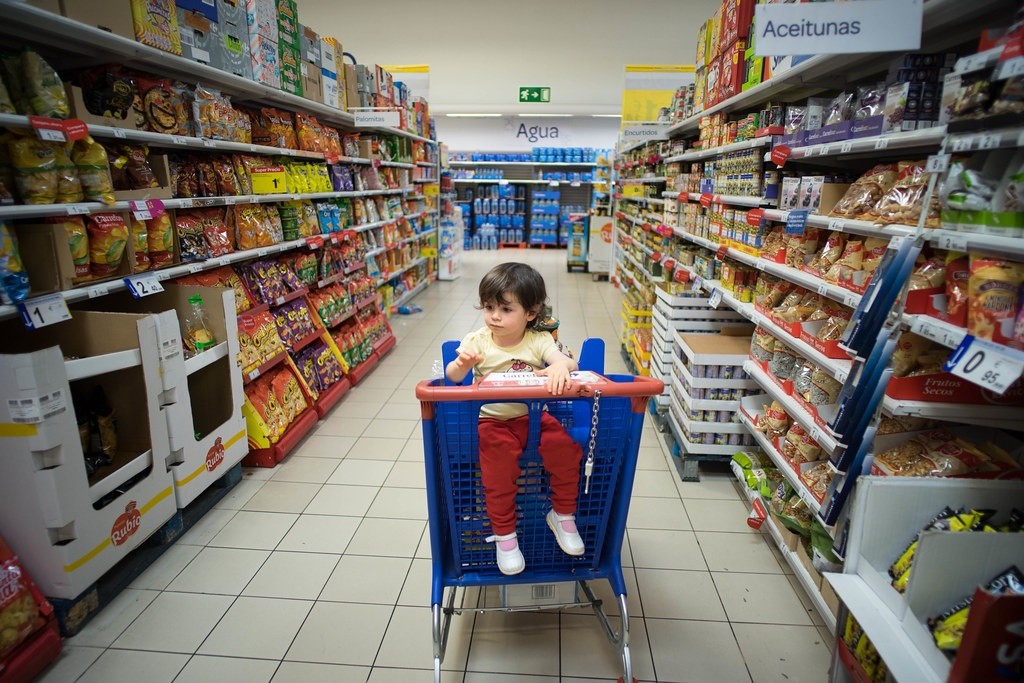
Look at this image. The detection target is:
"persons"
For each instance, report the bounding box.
[444,261,587,576]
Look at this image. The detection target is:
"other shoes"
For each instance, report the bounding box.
[485,532,525,575]
[546,507,584,555]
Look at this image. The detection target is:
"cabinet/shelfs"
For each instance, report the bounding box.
[439,165,464,280]
[237,261,397,467]
[448,161,609,184]
[0,0,440,321]
[609,0,1024,635]
[593,155,613,216]
[828,476,1024,683]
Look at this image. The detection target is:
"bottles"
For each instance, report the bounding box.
[532,198,559,236]
[474,198,515,214]
[500,228,522,242]
[538,169,543,180]
[473,236,497,250]
[474,168,504,179]
[561,205,583,236]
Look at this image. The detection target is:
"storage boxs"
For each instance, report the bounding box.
[66,284,249,509]
[692,0,834,114]
[0,310,176,601]
[27,0,437,141]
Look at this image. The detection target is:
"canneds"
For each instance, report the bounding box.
[670,340,760,447]
[663,148,821,301]
